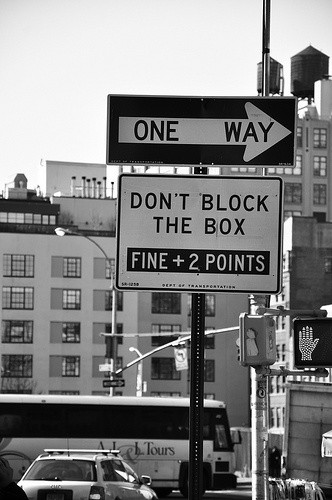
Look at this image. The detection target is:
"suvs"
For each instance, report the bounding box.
[17,448,159,500]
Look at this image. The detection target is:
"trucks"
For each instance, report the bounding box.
[279,379,332,492]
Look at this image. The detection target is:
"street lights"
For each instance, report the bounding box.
[54,226,118,396]
[128,346,143,397]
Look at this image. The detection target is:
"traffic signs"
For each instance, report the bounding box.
[102,379,126,388]
[106,94,298,169]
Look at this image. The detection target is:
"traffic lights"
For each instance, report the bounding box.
[238,312,278,367]
[291,317,332,368]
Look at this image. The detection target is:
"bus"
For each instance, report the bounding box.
[0,393,243,498]
